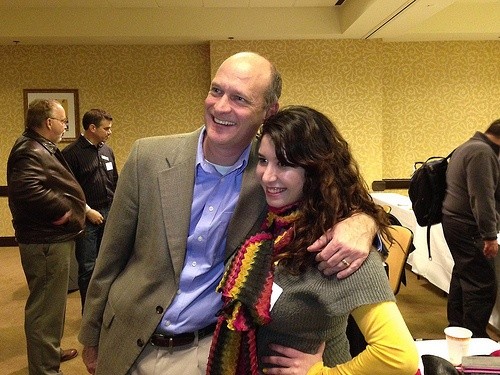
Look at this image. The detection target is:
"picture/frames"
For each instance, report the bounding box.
[22,88,80,142]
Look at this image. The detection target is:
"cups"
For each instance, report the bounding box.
[444,326,473,366]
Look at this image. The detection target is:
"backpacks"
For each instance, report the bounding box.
[408,146,457,261]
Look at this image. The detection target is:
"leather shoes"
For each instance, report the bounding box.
[60,348,78,362]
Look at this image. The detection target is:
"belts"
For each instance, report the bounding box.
[149,322,218,348]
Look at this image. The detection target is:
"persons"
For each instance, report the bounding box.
[441,119,500,338]
[77,51,281,375]
[7,98,86,375]
[60,108,119,316]
[204,105,420,375]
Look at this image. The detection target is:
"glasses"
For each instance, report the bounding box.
[46,117,67,124]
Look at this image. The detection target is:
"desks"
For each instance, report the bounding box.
[371,192,500,330]
[417,336,500,375]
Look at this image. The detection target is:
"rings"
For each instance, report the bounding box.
[342,259,350,266]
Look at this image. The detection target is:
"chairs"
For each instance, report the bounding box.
[382,224,413,295]
